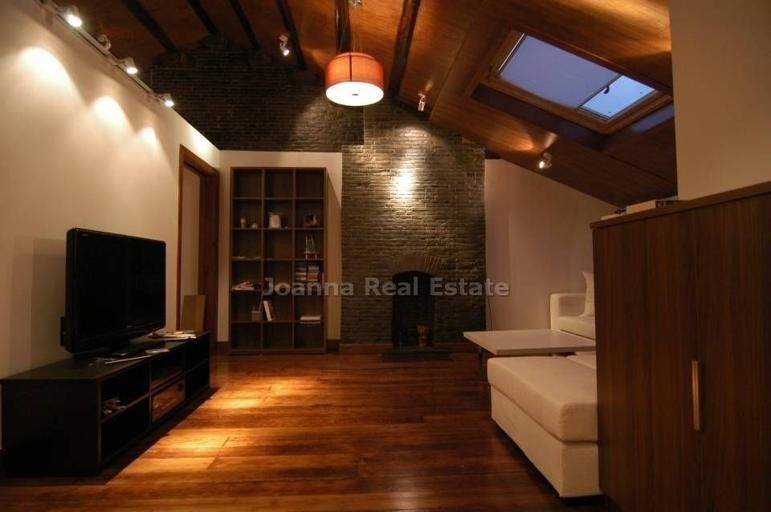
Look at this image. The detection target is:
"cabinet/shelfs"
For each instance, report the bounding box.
[229,165,330,355]
[1,334,211,480]
[582,182,769,512]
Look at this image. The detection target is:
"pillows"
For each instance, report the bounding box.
[578,274,595,317]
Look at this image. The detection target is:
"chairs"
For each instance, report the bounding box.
[546,290,596,342]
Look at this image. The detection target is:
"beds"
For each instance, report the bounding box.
[470,352,604,497]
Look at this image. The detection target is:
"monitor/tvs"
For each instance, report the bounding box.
[64,227,167,357]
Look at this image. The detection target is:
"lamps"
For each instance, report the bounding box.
[323,0,386,108]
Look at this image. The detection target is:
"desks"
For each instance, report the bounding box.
[463,326,596,367]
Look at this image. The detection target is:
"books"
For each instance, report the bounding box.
[295,262,321,291]
[231,279,258,292]
[625,199,683,215]
[301,311,322,321]
[263,299,276,322]
